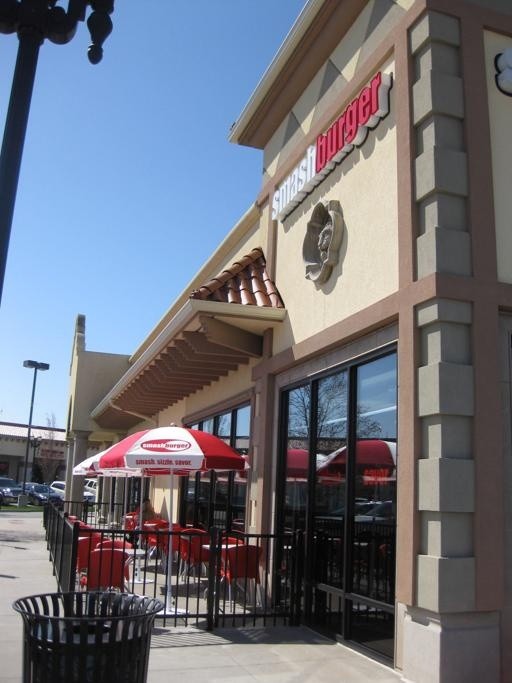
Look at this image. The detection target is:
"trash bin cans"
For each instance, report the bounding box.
[11,590,165,683]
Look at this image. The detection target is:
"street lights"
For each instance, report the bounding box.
[21,360,51,494]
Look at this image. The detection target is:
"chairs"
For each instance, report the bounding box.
[66,511,261,623]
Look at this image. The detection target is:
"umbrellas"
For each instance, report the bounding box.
[284,448,327,529]
[315,438,396,524]
[72,422,250,612]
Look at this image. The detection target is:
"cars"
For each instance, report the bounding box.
[311,498,396,542]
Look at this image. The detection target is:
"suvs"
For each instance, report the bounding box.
[0,477,99,506]
